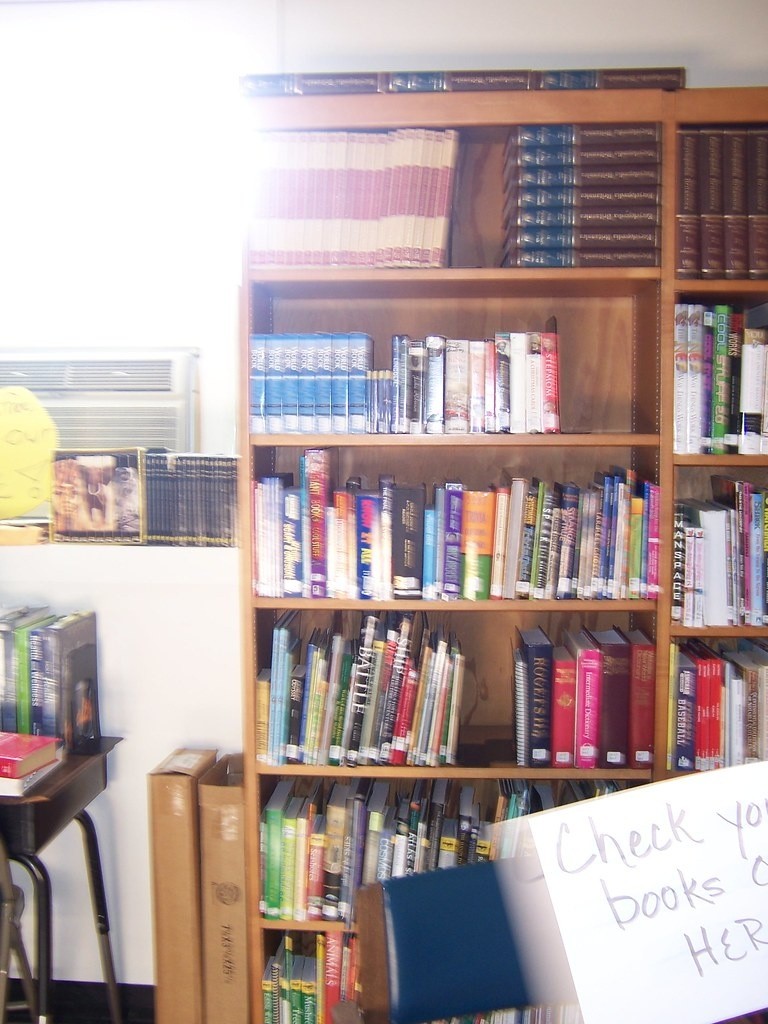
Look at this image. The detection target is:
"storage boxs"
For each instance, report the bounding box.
[486,760,768,1024]
[148,746,217,1024]
[197,753,252,1023]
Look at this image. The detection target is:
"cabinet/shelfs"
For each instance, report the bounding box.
[231,91,768,1024]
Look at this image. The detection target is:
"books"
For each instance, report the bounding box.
[239,66,689,94]
[248,331,565,434]
[669,473,768,630]
[255,613,654,770]
[260,779,622,1024]
[146,452,238,547]
[0,604,102,798]
[250,450,661,601]
[673,302,767,455]
[676,133,768,281]
[665,642,768,773]
[250,129,659,271]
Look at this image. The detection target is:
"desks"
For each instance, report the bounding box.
[0,736,125,1024]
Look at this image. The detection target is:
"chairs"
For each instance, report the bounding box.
[354,861,532,1024]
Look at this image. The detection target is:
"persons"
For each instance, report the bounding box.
[54,457,140,535]
